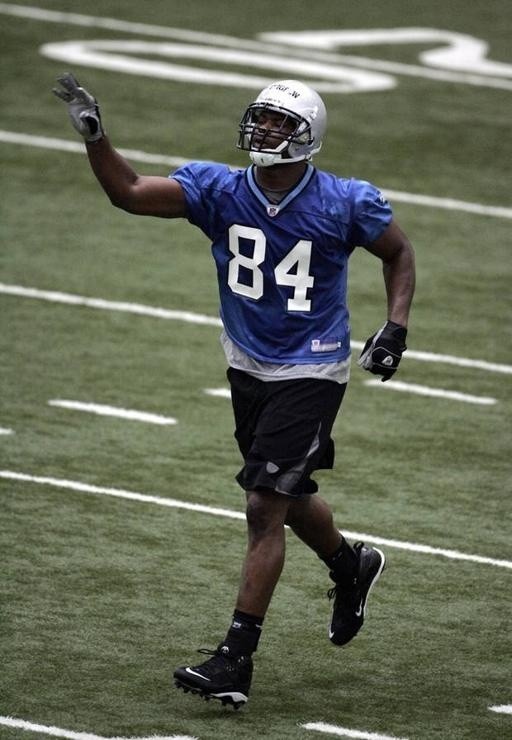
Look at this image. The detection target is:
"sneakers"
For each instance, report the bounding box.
[328,541,386,645]
[172,641,253,710]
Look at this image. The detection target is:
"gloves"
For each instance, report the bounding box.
[356,320,407,382]
[53,71,105,144]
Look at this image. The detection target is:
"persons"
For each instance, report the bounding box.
[50,71,416,709]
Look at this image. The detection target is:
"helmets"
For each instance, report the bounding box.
[235,80,327,167]
